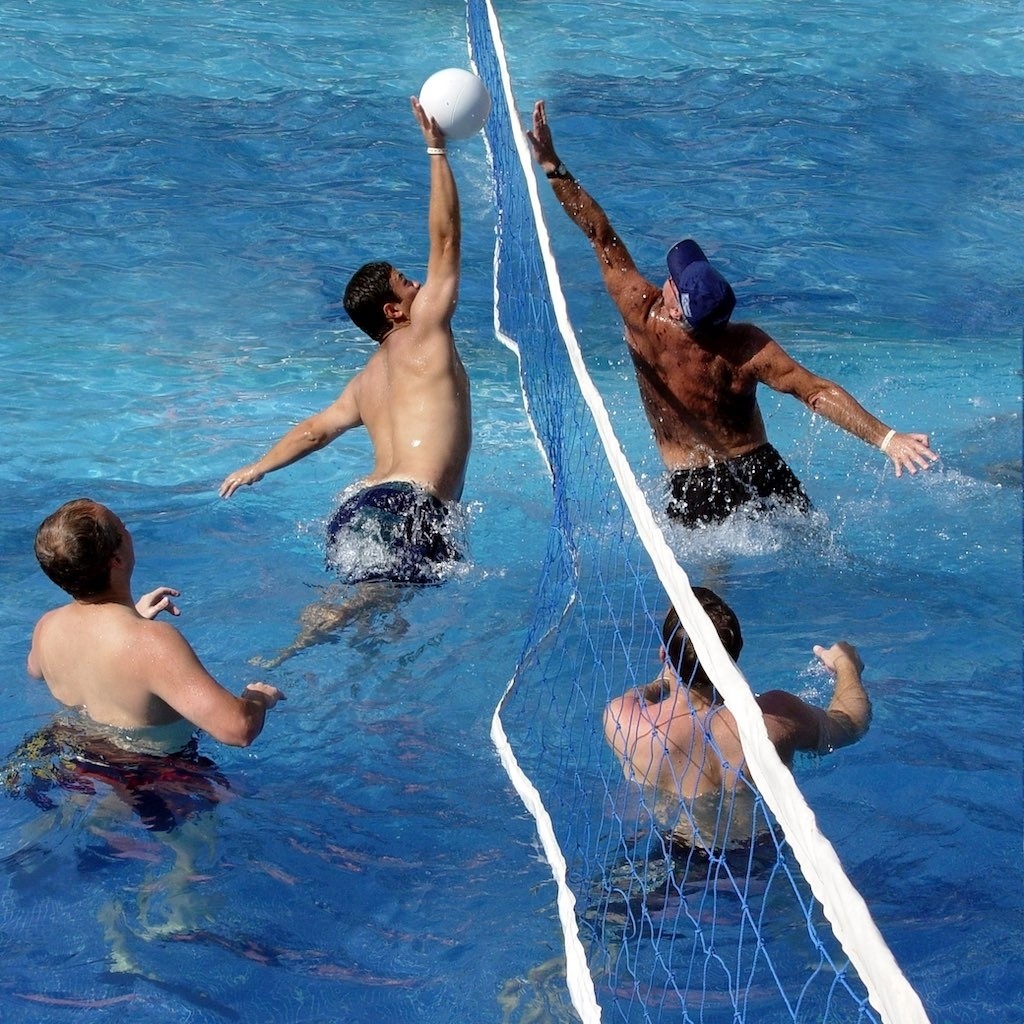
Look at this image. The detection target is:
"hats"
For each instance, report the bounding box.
[666,238,736,333]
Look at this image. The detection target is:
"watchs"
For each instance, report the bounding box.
[546,162,567,178]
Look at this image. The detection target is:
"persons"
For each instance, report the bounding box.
[216,96,473,560]
[31,499,288,748]
[603,586,873,799]
[526,99,941,527]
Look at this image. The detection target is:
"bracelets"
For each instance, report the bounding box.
[426,147,447,154]
[881,429,896,452]
[654,676,670,683]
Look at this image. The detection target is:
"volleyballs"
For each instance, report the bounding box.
[418,66,490,140]
